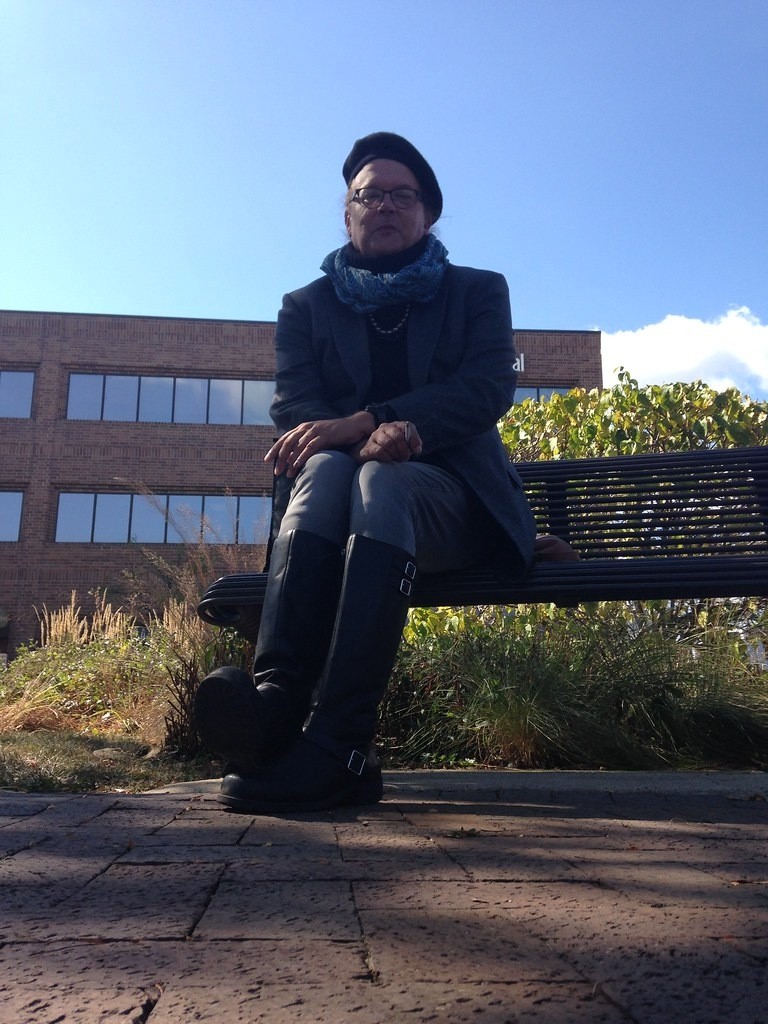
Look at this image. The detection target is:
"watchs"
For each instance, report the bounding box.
[364,401,388,429]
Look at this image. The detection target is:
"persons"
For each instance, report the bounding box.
[190,131,536,816]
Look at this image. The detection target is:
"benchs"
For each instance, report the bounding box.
[194,439,768,645]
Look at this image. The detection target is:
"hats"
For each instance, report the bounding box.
[341,131,442,224]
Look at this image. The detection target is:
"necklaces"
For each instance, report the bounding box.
[368,300,412,333]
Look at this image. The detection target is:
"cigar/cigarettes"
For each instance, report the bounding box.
[404,420,411,444]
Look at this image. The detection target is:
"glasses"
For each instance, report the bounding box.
[351,186,421,209]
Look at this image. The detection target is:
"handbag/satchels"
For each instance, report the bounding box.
[533,534,580,561]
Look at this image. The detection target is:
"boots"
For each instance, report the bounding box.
[217,534,421,811]
[194,526,341,767]
